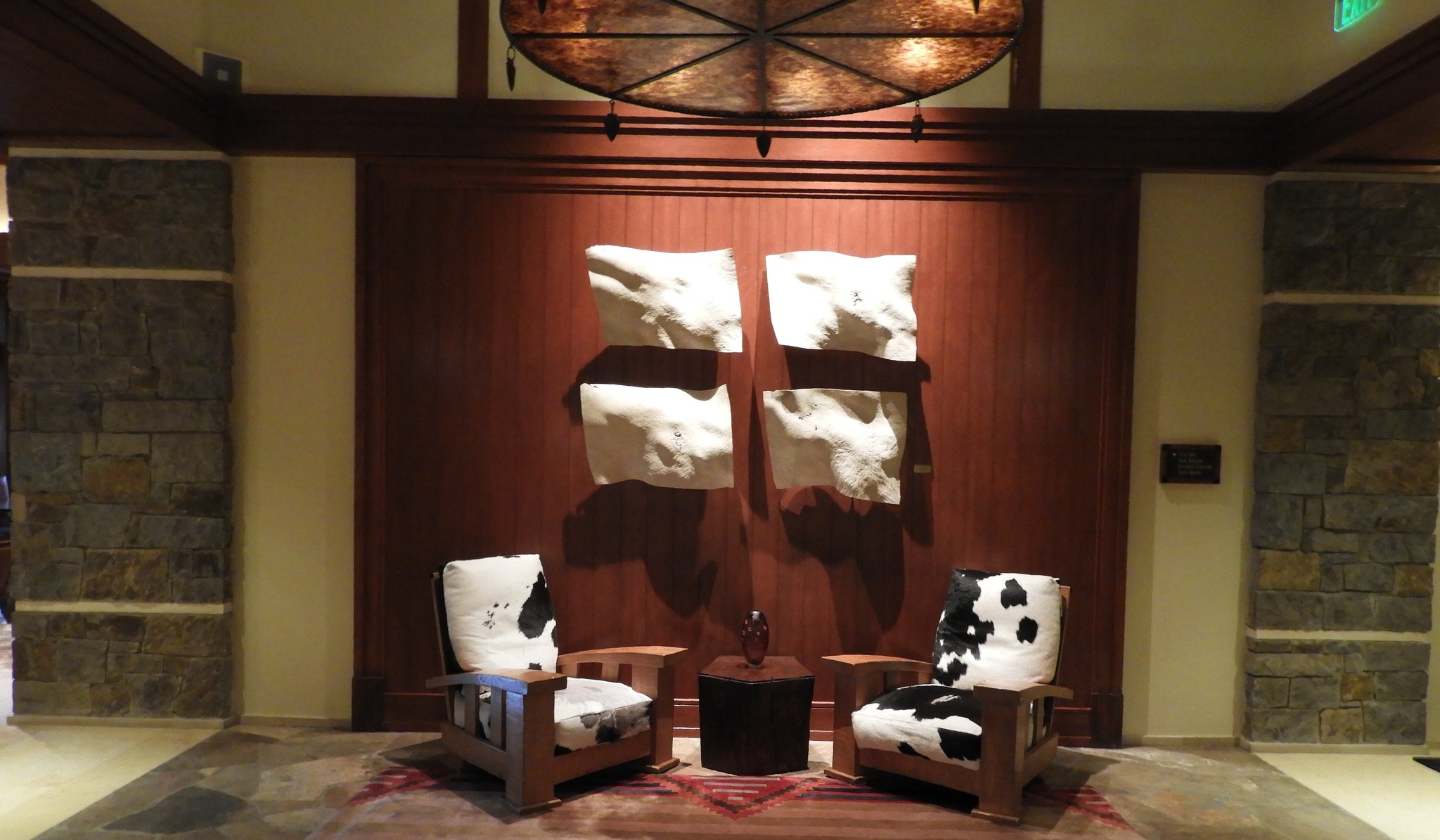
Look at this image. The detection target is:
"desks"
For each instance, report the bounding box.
[698,655,815,776]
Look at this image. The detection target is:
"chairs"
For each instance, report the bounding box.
[426,553,689,818]
[819,569,1077,825]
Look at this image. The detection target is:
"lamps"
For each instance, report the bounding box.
[498,0,1028,157]
